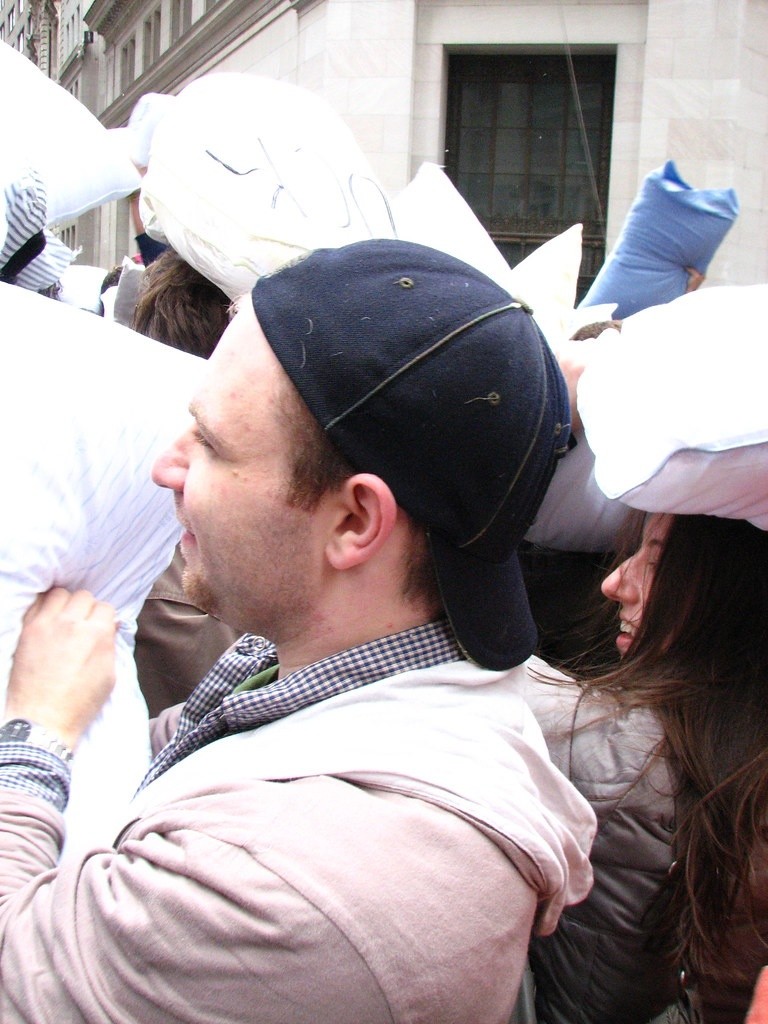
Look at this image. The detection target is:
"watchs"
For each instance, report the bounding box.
[0,718,82,770]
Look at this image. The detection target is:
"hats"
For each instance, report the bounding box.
[253,238,574,672]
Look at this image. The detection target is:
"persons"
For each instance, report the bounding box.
[1,30,710,730]
[493,321,768,1024]
[1,238,602,1024]
